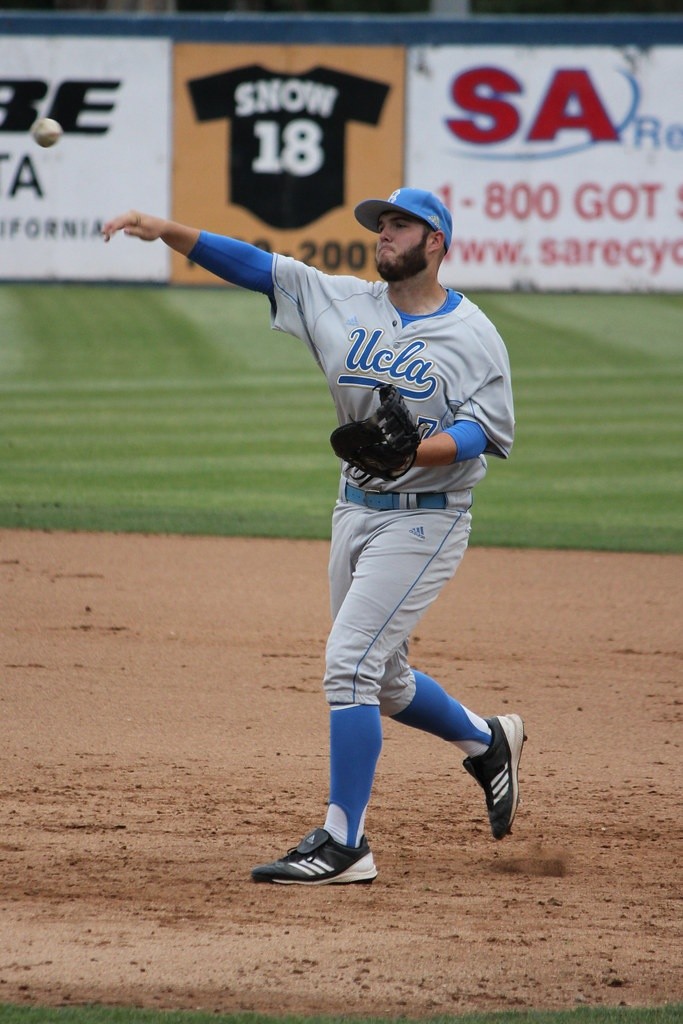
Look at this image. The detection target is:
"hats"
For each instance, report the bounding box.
[355,187,453,252]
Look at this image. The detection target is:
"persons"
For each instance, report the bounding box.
[103,188,526,888]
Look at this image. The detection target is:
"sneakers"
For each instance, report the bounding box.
[463,714,525,840]
[252,827,378,886]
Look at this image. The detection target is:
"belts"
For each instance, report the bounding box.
[345,483,446,510]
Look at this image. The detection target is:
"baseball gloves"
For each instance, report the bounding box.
[328,379,422,482]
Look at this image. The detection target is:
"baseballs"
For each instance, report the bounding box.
[30,116,64,148]
